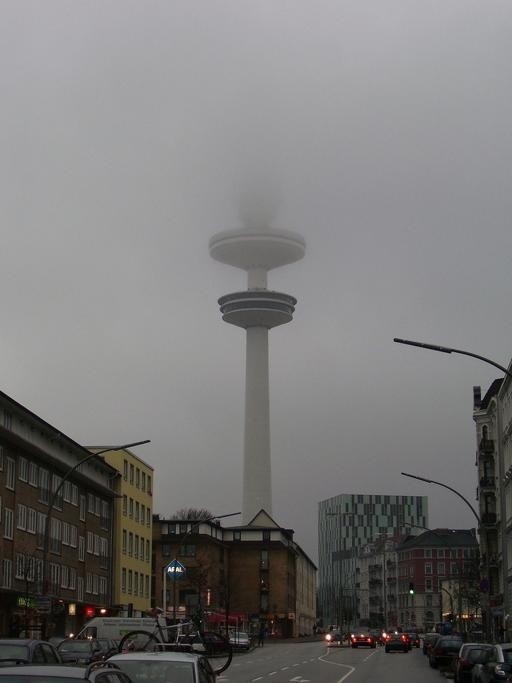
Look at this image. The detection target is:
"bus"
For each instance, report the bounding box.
[425,621,452,634]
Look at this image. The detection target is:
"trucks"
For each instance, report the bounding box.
[74,616,170,651]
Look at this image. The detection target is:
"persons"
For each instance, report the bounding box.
[258,629,264,648]
[313,624,317,635]
[330,624,333,632]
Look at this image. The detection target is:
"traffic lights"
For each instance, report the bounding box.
[408,581,414,594]
[449,614,453,623]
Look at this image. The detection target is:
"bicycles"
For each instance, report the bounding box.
[118,606,234,675]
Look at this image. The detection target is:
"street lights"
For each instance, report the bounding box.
[172,511,242,621]
[391,335,512,382]
[403,521,470,641]
[400,471,491,643]
[344,588,384,631]
[41,438,151,640]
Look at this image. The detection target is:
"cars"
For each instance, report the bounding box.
[228,632,252,649]
[1,637,217,683]
[329,630,511,682]
